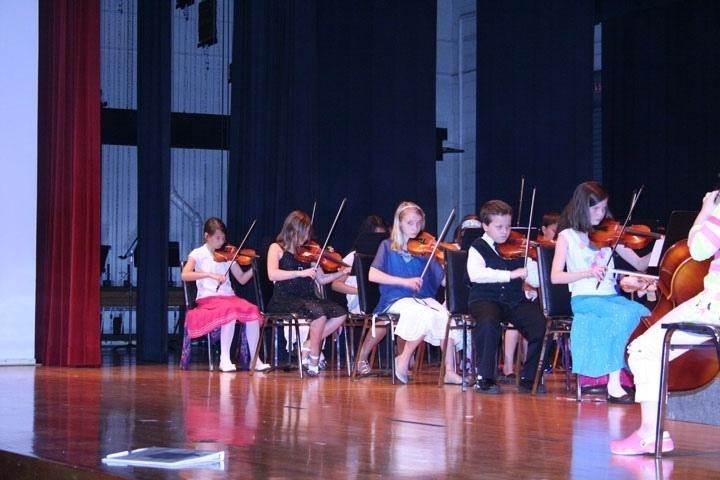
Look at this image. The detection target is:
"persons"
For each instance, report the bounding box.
[331,216,388,375]
[179,217,272,372]
[455,214,480,248]
[466,200,546,394]
[541,212,559,241]
[267,209,346,376]
[551,182,651,403]
[608,187,719,456]
[368,202,462,384]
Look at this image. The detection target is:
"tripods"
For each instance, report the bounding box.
[112,286,136,354]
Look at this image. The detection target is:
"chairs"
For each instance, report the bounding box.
[177,260,250,375]
[250,252,328,381]
[329,252,422,386]
[525,245,587,404]
[652,321,720,461]
[435,249,523,394]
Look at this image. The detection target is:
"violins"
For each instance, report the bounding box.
[495,231,557,261]
[407,231,458,263]
[214,243,262,266]
[293,240,351,271]
[588,218,661,249]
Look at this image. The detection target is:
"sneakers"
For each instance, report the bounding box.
[518,378,547,394]
[218,363,237,372]
[248,362,272,371]
[475,379,505,394]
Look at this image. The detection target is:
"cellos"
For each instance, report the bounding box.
[624,191,720,382]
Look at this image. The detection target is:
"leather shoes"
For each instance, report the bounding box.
[443,373,463,384]
[499,373,516,385]
[606,392,636,404]
[394,356,408,384]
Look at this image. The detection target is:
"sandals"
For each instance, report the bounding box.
[609,429,675,455]
[351,360,372,375]
[300,339,329,377]
